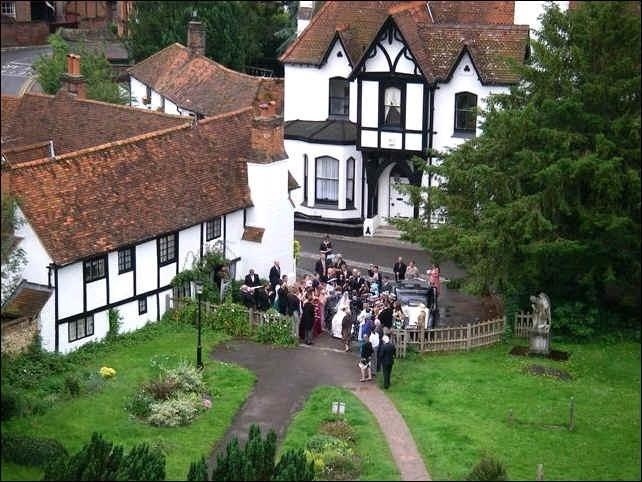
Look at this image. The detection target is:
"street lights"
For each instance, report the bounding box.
[195,280,204,369]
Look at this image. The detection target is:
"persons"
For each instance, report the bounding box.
[239,235,441,389]
